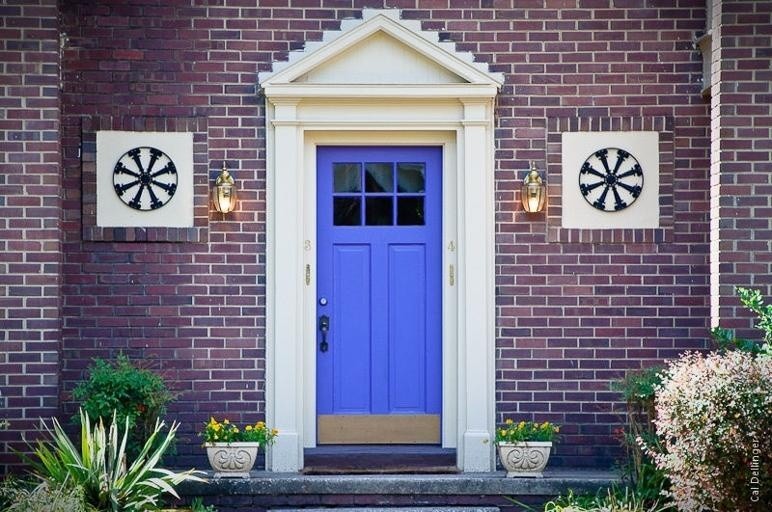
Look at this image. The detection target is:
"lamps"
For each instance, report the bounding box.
[213,161,237,214]
[522,161,547,216]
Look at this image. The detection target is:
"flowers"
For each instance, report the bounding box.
[493,419,561,442]
[198,415,278,448]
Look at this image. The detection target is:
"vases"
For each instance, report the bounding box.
[496,442,551,478]
[205,442,259,480]
